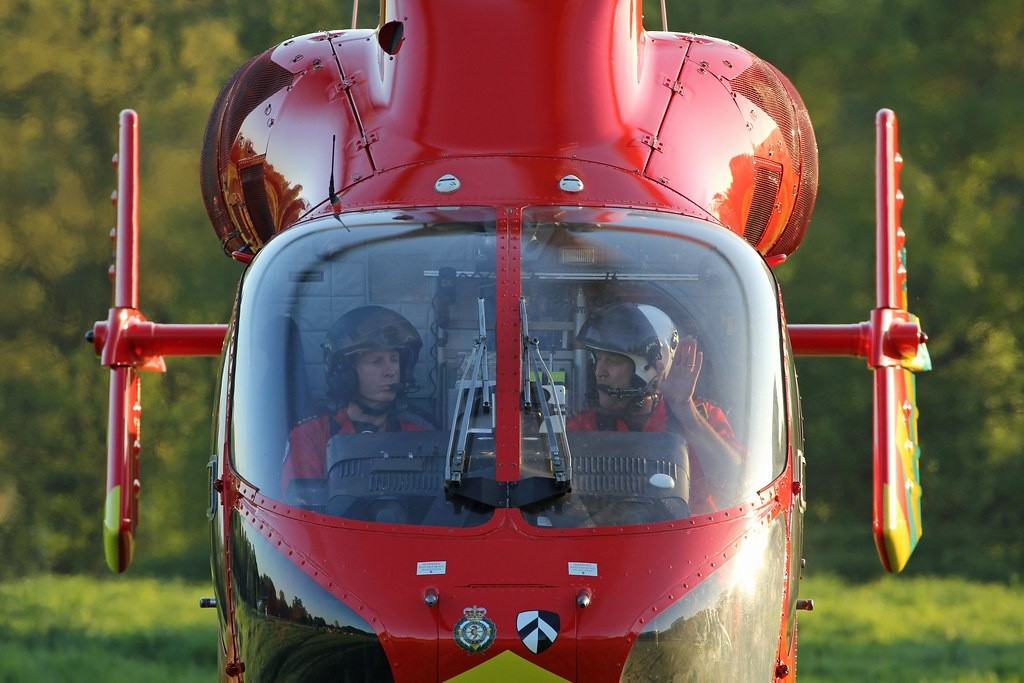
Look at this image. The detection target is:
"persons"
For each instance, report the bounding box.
[564,302,751,515]
[282,306,439,496]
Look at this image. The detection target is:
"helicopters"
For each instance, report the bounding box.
[88,0,934,683]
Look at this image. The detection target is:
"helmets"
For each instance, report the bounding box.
[579,303,680,393]
[323,305,422,397]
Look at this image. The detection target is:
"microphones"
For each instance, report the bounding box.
[596,383,655,397]
[390,380,422,394]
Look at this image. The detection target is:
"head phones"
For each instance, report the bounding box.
[319,344,415,400]
